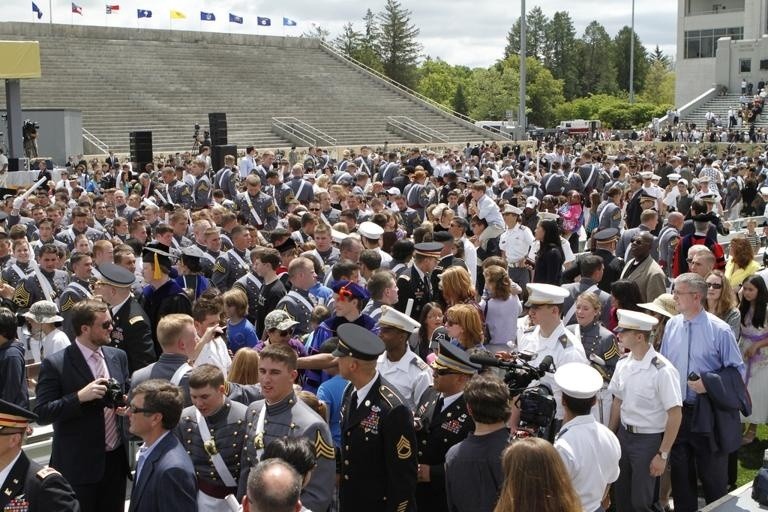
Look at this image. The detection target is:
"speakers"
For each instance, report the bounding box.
[211,143,238,173]
[30,157,53,170]
[209,112,228,145]
[8,157,29,171]
[129,131,153,173]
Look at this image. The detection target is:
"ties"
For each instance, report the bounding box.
[347,391,358,422]
[433,398,444,420]
[93,352,120,451]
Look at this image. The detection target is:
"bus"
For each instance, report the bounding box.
[472,118,519,134]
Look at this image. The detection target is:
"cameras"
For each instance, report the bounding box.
[101,378,123,407]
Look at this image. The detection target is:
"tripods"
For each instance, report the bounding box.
[192,141,201,155]
[23,132,38,157]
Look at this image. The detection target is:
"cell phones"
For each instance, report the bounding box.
[215,326,227,337]
[688,371,699,381]
[524,259,535,270]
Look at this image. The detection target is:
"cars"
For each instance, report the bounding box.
[527,124,545,137]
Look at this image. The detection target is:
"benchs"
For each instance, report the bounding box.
[678,92,768,141]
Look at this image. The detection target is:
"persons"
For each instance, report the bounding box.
[1,79,767,511]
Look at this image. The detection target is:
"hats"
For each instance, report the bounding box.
[552,359,606,403]
[94,260,137,289]
[611,307,662,334]
[424,340,484,376]
[259,309,302,333]
[0,399,41,429]
[376,303,424,336]
[329,321,385,362]
[19,299,64,325]
[141,144,767,311]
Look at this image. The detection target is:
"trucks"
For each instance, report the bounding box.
[555,118,602,137]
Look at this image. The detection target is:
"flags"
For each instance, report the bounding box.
[138,8,152,17]
[283,17,296,26]
[229,13,243,24]
[201,11,215,21]
[72,2,83,15]
[106,4,119,15]
[258,16,270,26]
[33,1,43,20]
[170,9,185,19]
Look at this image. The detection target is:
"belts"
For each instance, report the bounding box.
[620,418,668,435]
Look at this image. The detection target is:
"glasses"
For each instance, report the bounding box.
[90,320,116,329]
[274,328,295,337]
[125,404,156,414]
